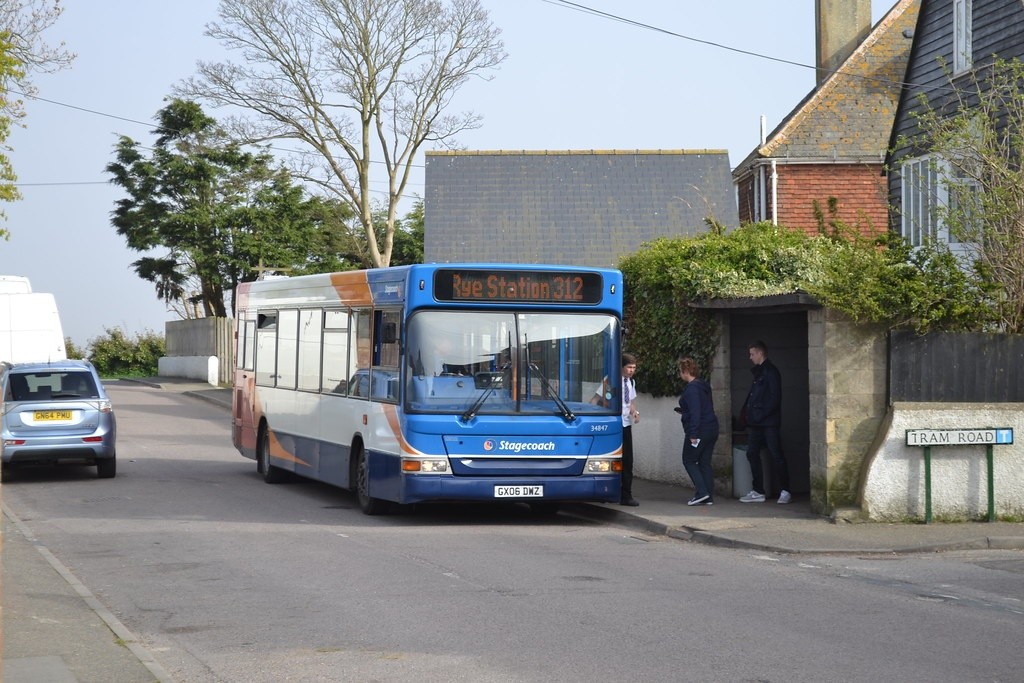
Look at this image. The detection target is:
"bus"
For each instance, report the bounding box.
[230,260,629,517]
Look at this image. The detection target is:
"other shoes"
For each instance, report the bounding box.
[687,491,710,506]
[699,499,714,505]
[620,489,639,507]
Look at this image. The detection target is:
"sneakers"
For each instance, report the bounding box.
[739,490,766,503]
[777,490,792,504]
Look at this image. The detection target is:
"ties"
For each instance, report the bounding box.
[624,378,629,402]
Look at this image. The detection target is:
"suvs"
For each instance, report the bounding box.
[0,358,120,483]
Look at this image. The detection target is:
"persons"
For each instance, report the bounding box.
[677,358,719,506]
[738,342,794,505]
[589,354,640,507]
